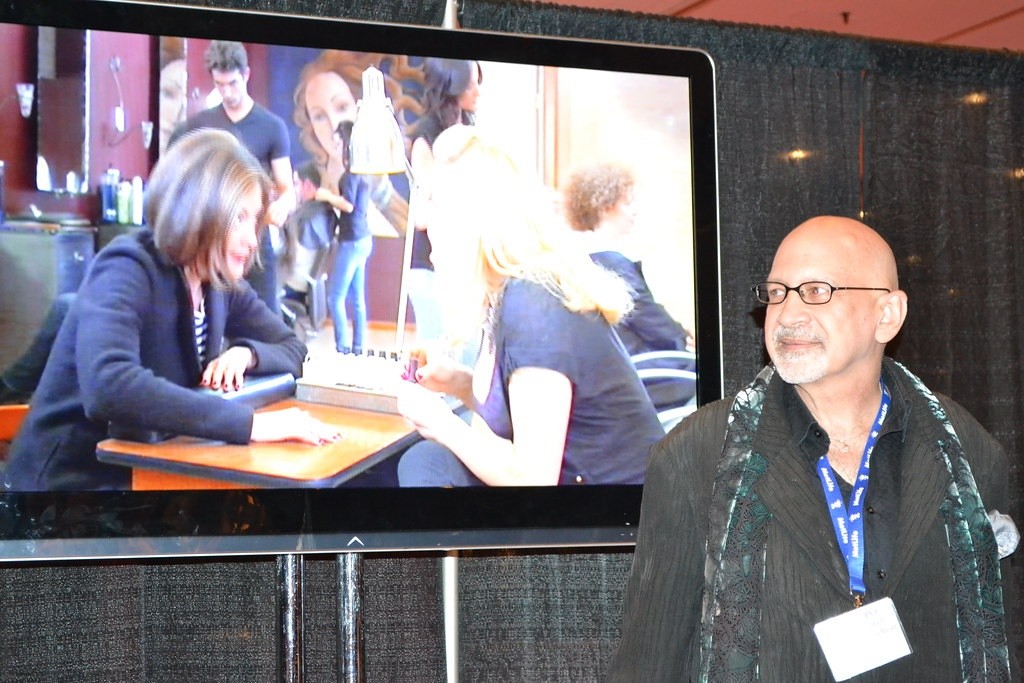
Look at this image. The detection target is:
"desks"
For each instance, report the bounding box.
[96,387,469,491]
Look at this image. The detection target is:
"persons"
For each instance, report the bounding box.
[3,129,340,493]
[397,125,664,486]
[614,214,1024,683]
[157,40,694,410]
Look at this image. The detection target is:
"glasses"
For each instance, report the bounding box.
[748,281,892,306]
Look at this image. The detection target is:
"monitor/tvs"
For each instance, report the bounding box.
[0,0,724,562]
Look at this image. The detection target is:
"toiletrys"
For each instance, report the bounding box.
[98,167,143,226]
[16,83,35,117]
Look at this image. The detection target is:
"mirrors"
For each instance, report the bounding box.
[35,75,89,194]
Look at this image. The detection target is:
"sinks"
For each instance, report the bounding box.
[6,212,80,223]
